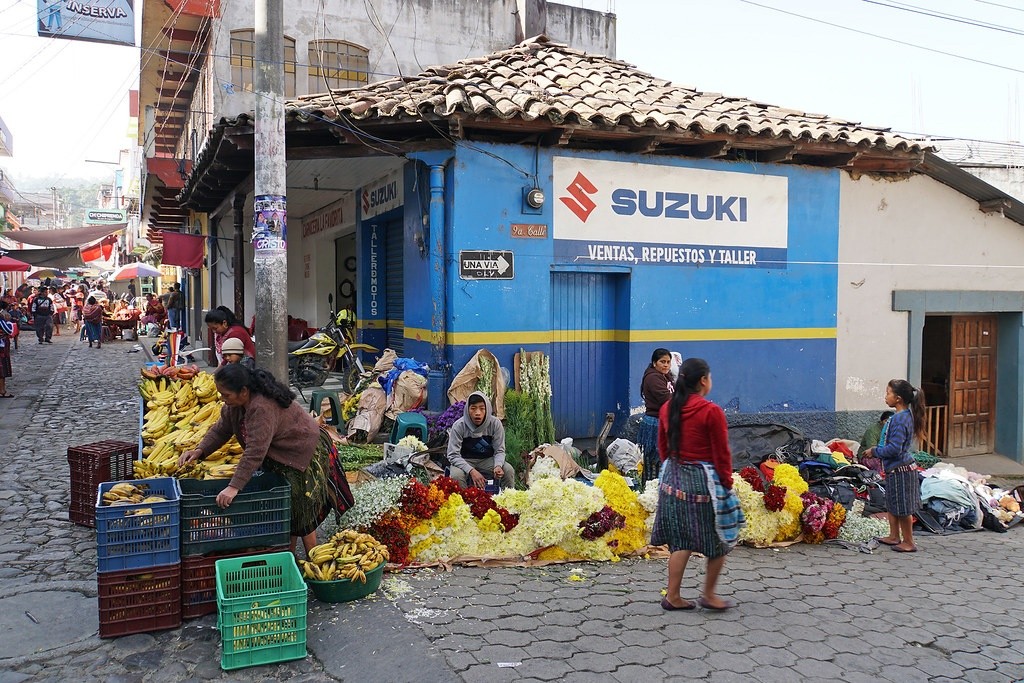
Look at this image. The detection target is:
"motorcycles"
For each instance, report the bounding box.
[287,293,381,396]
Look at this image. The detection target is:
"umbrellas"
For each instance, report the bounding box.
[28,268,67,279]
[109,261,165,310]
[0,255,32,272]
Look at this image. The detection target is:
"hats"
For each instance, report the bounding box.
[221,338,244,354]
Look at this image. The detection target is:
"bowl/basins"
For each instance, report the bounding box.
[303,559,385,603]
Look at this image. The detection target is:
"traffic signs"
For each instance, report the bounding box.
[458,250,516,280]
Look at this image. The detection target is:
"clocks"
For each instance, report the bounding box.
[344,256,356,272]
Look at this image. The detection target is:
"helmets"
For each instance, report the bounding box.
[336,308,356,326]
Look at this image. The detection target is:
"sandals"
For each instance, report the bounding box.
[0,391,15,398]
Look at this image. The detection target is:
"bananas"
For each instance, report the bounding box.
[234,600,297,649]
[102,482,171,619]
[133,364,244,480]
[298,528,390,584]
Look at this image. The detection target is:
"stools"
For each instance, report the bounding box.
[388,412,428,444]
[101,326,113,342]
[80,325,87,341]
[310,390,347,435]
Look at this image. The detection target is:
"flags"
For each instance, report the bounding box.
[160,232,205,269]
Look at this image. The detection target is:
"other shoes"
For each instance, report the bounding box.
[661,598,696,610]
[45,339,53,344]
[892,543,917,552]
[89,343,93,347]
[97,342,101,349]
[39,339,42,344]
[700,598,731,610]
[53,333,60,336]
[878,537,901,545]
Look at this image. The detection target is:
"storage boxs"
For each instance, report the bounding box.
[68,439,308,670]
[444,464,499,493]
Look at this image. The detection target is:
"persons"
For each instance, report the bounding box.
[179,361,354,562]
[650,357,745,610]
[0,300,15,398]
[636,347,676,487]
[446,391,515,492]
[128,279,136,307]
[205,306,255,375]
[31,287,54,344]
[862,378,927,552]
[83,295,102,348]
[221,337,255,370]
[140,282,185,334]
[0,277,129,349]
[858,411,895,472]
